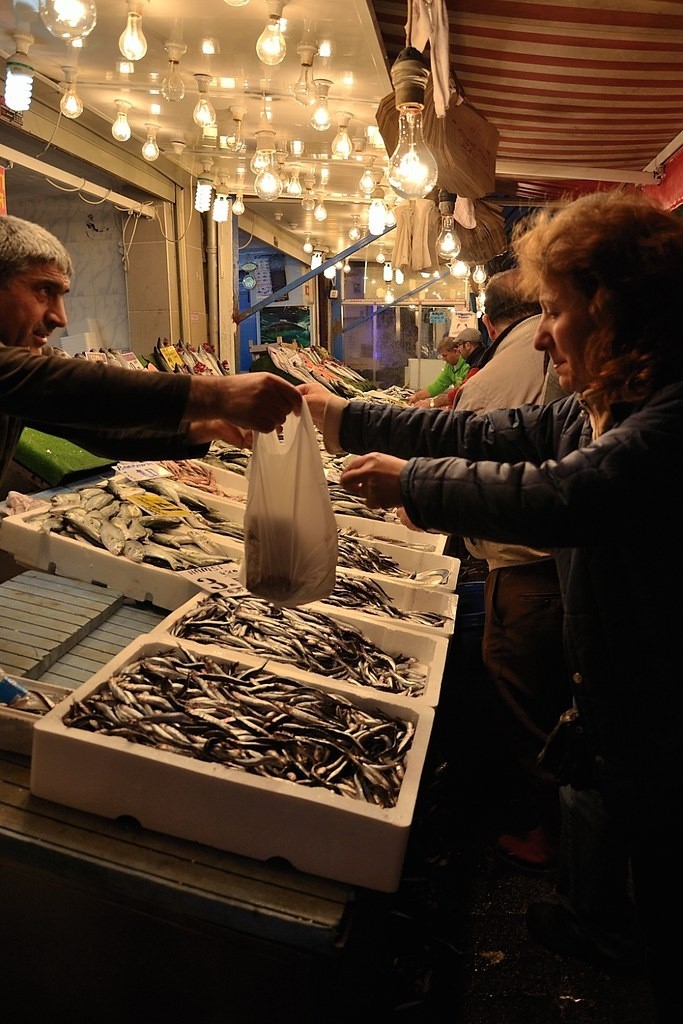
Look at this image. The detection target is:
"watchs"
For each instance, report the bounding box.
[430,399,434,408]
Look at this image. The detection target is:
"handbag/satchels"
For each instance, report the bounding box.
[242,392,338,607]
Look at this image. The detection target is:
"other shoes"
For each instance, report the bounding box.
[527,903,643,973]
[496,829,557,872]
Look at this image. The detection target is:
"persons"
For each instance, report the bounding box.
[409,269,573,788]
[0,215,303,504]
[299,195,682,1024]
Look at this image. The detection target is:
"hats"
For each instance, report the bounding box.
[452,328,484,344]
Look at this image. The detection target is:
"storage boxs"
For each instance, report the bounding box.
[1,383,461,893]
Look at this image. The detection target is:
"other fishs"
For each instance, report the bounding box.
[26,336,452,810]
